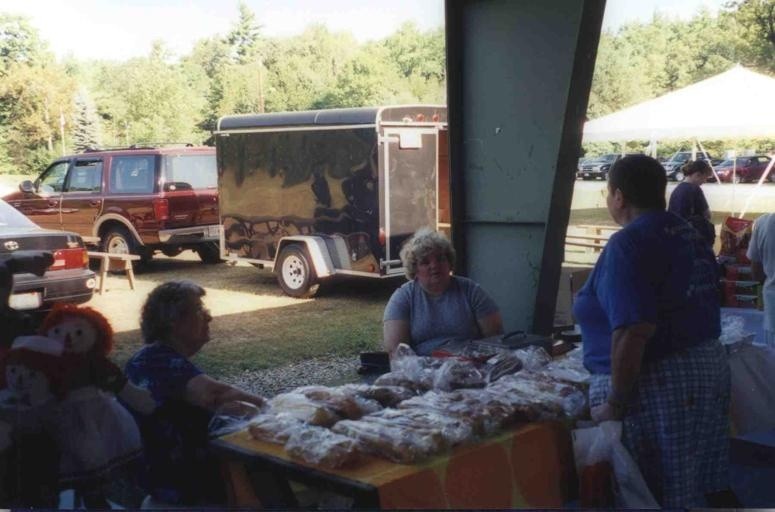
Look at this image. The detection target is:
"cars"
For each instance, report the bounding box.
[0,194,98,309]
[575,145,775,188]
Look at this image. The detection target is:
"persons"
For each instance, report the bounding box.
[119,282,321,512]
[668,160,716,248]
[744,212,775,345]
[570,154,744,511]
[379,228,502,356]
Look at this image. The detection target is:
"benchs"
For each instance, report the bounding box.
[88,251,142,294]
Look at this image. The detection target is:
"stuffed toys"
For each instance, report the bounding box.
[0,335,70,512]
[41,301,161,512]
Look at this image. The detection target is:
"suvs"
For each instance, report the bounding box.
[1,138,229,277]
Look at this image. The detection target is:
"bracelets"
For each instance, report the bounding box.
[603,398,630,412]
